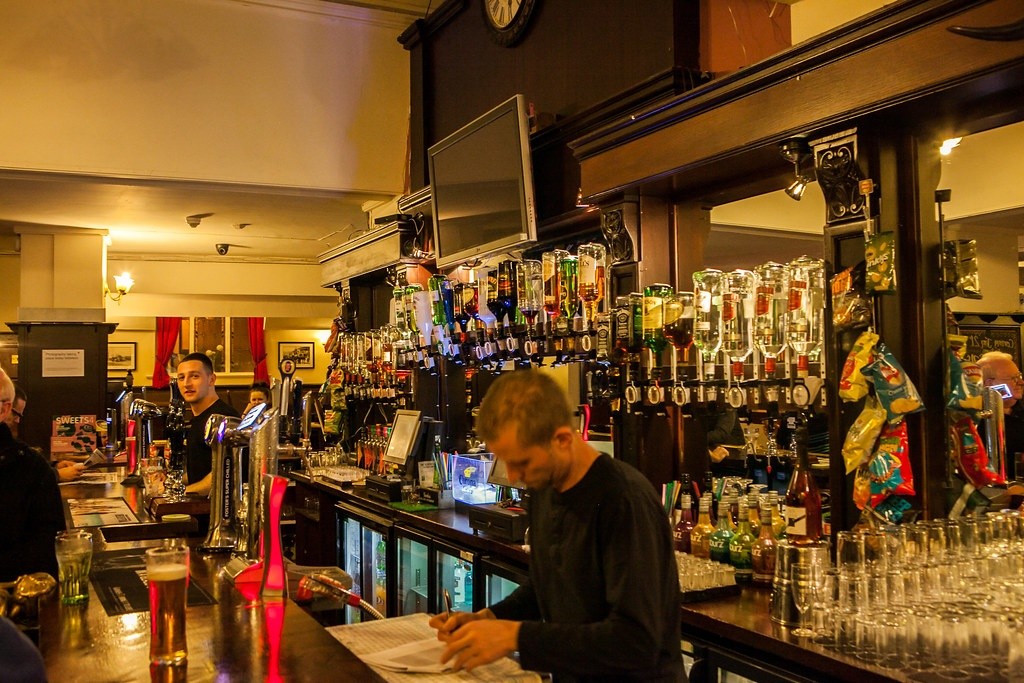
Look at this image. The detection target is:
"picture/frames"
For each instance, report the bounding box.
[278,341,314,369]
[107,342,137,370]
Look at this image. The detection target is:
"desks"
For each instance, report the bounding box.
[38,429,388,683]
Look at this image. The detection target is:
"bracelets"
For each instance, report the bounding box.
[243,413,246,416]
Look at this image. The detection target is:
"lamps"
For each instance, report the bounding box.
[778,140,808,201]
[104,269,134,300]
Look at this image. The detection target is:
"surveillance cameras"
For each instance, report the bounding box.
[187,217,200,228]
[216,244,229,255]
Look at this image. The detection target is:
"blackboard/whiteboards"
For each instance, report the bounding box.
[961,330,1018,367]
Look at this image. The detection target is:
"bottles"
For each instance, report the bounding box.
[276,241,831,598]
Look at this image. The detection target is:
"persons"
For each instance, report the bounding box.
[177,353,250,496]
[0,367,67,593]
[707,407,747,444]
[429,369,687,683]
[975,351,1024,480]
[3,388,88,482]
[241,382,272,425]
[803,385,829,454]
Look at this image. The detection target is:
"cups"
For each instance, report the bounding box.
[790,507,1024,683]
[146,545,191,667]
[53,528,93,607]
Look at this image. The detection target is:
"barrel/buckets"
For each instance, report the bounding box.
[770,539,831,627]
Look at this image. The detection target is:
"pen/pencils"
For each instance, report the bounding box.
[445,589,452,617]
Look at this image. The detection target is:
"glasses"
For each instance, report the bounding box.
[11,408,24,422]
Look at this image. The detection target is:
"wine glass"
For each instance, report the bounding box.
[789,563,821,637]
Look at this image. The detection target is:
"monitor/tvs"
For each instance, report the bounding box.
[383,409,425,481]
[427,93,538,270]
